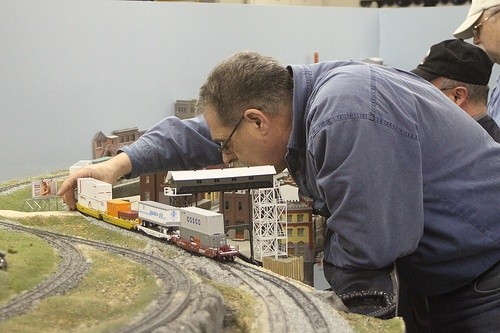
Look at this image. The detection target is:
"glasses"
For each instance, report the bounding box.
[472,9,500,37]
[218,105,263,160]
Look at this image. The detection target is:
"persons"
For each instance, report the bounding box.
[56,50,500,333]
[410,37,500,146]
[452,0,500,128]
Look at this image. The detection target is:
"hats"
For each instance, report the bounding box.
[410,37,494,84]
[453,0,500,39]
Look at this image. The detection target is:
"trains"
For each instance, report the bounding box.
[73,177,240,263]
